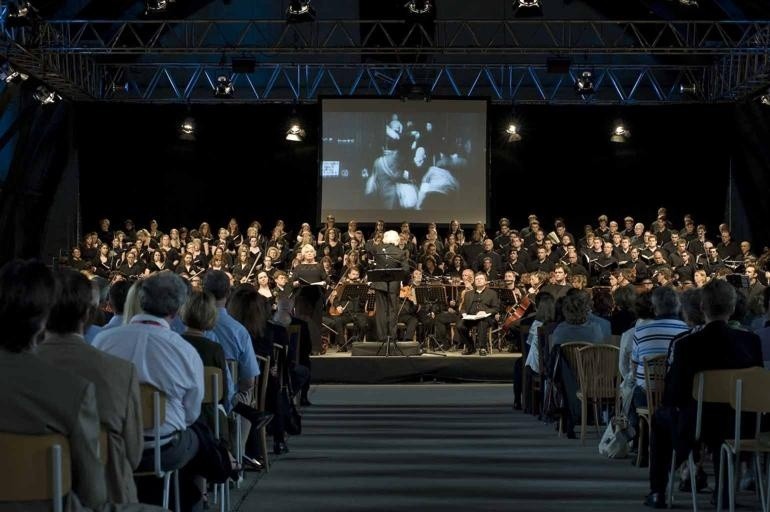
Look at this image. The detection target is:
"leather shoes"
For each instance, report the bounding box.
[644,494,664,508]
[273,444,288,454]
[479,349,486,356]
[462,349,476,355]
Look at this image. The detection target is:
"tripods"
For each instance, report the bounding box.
[336,300,362,352]
[367,281,405,356]
[415,301,446,354]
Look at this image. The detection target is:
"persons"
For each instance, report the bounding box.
[363,111,471,212]
[501,206,769,511]
[2,207,336,512]
[313,213,504,355]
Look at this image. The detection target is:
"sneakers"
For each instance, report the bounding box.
[252,412,275,428]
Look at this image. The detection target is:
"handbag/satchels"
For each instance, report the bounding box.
[540,379,568,423]
[279,392,302,436]
[598,416,634,459]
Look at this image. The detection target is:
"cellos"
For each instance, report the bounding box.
[502,271,546,331]
